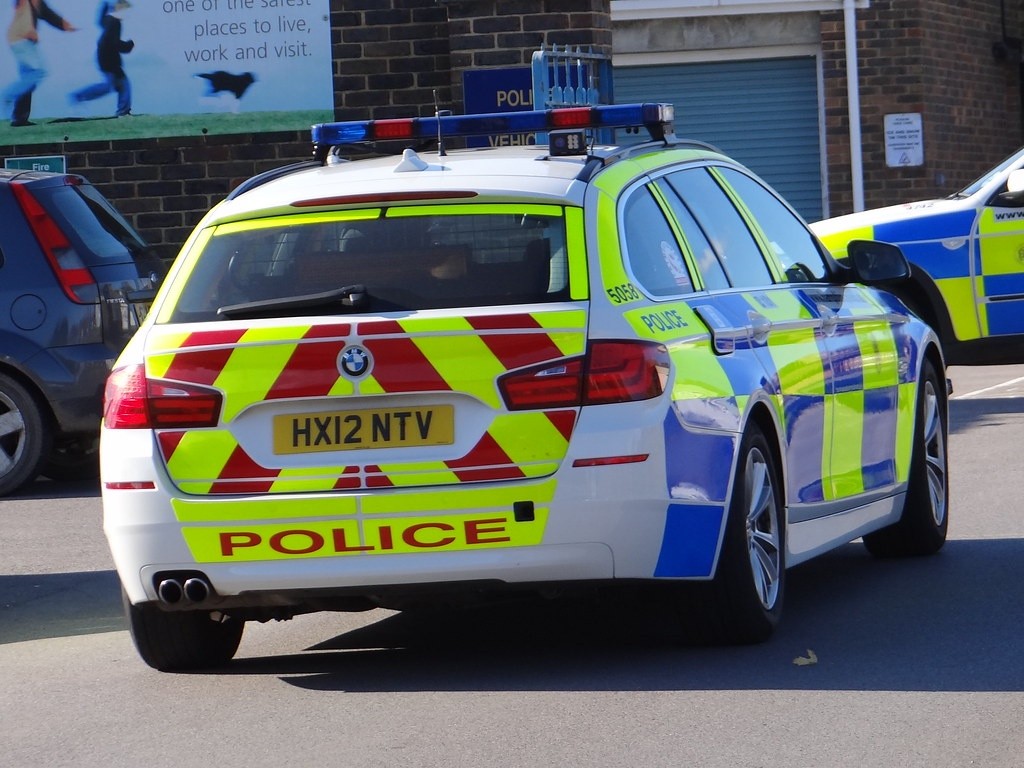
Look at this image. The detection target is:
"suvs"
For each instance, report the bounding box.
[0,168,170,498]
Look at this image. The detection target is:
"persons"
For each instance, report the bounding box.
[0,0,79,126]
[65,0,137,119]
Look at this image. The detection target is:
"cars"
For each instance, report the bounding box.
[100,101,949,674]
[809,147,1024,367]
